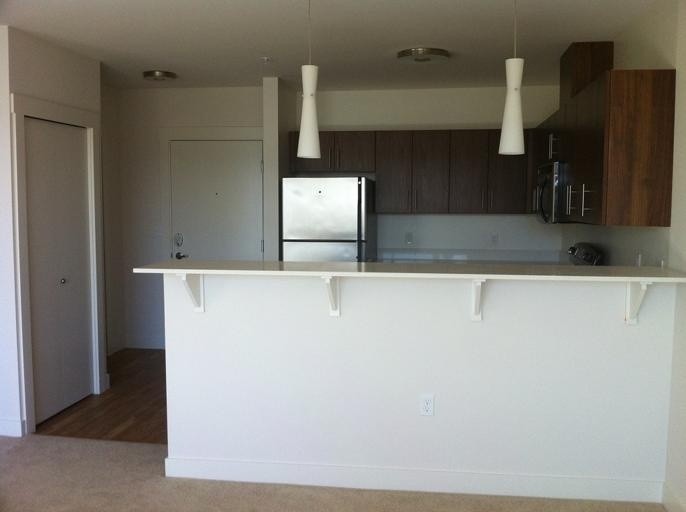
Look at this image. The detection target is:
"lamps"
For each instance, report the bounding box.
[295,0,321,160]
[498,1,526,156]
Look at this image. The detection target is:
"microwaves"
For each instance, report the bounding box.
[537,161,558,224]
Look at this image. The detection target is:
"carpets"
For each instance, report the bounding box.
[0,434,667,512]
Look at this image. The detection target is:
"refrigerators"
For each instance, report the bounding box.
[280,174,380,259]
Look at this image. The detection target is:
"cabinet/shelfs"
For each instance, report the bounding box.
[533,105,561,161]
[566,67,677,228]
[451,128,528,215]
[377,130,450,215]
[289,130,376,174]
